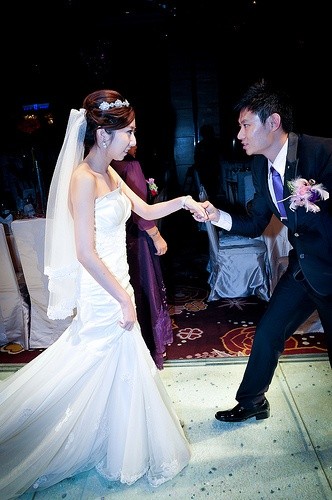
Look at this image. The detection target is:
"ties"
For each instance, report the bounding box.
[271,166,287,222]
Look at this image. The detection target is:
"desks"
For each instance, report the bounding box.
[222,176,239,204]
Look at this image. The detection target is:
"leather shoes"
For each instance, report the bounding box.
[215,399,270,422]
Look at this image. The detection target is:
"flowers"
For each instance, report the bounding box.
[273,175,330,215]
[146,178,159,198]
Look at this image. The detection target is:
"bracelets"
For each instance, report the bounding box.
[148,226,158,237]
[181,195,192,209]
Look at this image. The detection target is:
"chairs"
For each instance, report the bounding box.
[11,218,77,352]
[198,185,273,303]
[0,223,30,351]
[152,187,168,236]
[30,145,46,214]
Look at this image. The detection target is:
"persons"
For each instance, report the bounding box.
[44,90,210,488]
[190,83,332,422]
[109,144,174,370]
